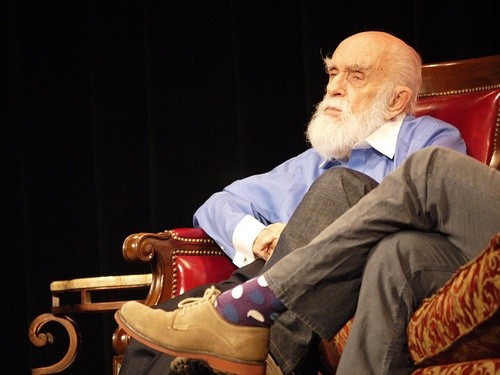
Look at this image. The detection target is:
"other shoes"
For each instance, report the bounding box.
[114,288,269,375]
[168,353,285,375]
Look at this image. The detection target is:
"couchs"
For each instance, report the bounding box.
[112,55,500,375]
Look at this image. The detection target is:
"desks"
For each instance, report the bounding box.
[27,274,155,375]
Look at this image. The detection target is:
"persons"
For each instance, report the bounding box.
[116,31,471,375]
[111,146,500,374]
